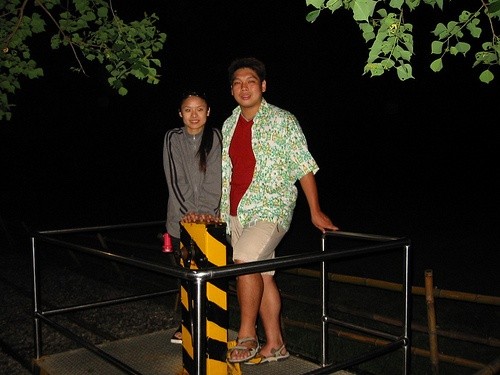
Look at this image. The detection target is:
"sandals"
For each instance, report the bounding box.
[229,335,260,362]
[244,344,290,364]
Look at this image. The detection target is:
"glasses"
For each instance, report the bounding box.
[178,90,209,98]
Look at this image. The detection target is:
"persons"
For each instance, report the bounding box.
[162,89,224,344]
[218,58,342,368]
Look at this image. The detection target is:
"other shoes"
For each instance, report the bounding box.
[170,325,183,344]
